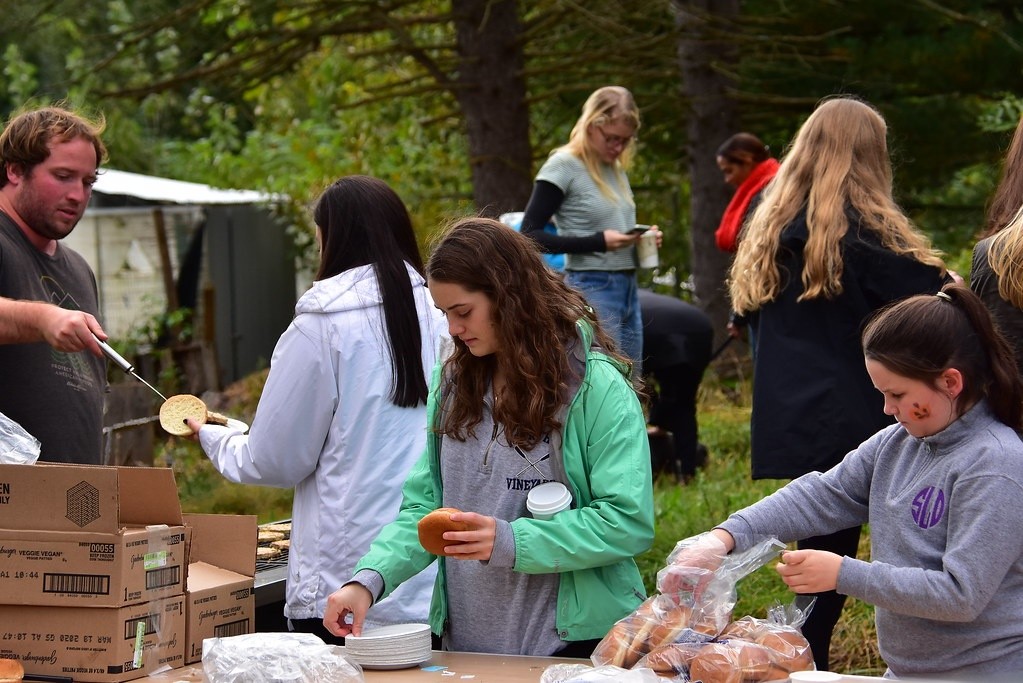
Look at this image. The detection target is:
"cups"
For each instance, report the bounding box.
[636,231,659,268]
[789,671,841,683]
[526,482,572,520]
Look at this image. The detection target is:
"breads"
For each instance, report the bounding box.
[594,596,814,683]
[0,659,25,683]
[418,508,476,556]
[159,395,208,436]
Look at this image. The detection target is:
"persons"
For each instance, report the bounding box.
[637,288,713,485]
[519,87,663,394]
[499,212,564,273]
[714,132,780,339]
[322,218,655,658]
[664,286,1023,683]
[184,174,457,646]
[726,100,964,670]
[0,108,108,464]
[969,121,1023,439]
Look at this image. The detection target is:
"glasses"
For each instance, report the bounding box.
[598,126,634,146]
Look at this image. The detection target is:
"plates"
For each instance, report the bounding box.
[224,417,249,433]
[345,623,431,671]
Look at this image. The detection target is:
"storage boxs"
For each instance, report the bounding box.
[0,461,261,683]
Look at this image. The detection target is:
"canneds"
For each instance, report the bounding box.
[637,230,659,269]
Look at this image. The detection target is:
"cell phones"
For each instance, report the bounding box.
[624,224,650,235]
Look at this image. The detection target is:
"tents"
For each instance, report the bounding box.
[59,163,297,396]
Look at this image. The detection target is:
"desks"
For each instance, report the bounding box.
[124,649,681,683]
